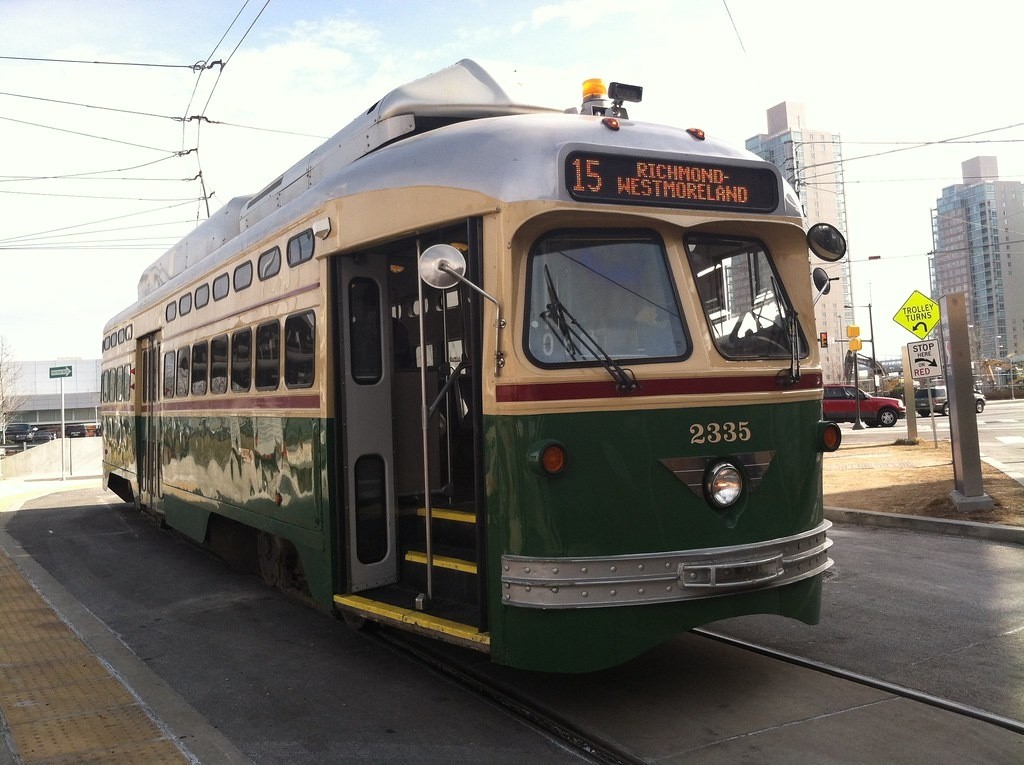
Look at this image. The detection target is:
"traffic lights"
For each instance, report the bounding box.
[820,332,828,348]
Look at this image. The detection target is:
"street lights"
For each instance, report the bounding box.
[995,335,1003,388]
[845,304,878,396]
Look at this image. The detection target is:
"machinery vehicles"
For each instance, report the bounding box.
[844,351,920,402]
[984,357,1024,389]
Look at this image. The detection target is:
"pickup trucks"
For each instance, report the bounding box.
[5,423,34,443]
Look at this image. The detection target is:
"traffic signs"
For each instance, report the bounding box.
[50,365,71,377]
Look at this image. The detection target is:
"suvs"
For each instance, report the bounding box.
[821,386,907,429]
[915,384,987,416]
[65,424,87,437]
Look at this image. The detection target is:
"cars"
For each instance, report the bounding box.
[33,431,56,443]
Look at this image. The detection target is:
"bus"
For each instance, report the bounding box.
[100,60,851,678]
